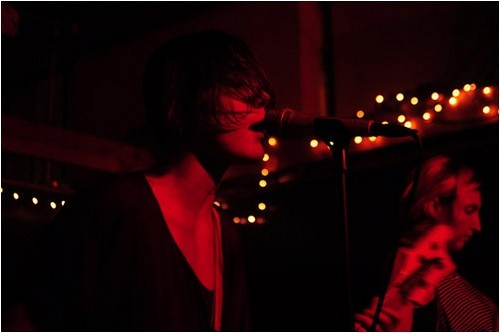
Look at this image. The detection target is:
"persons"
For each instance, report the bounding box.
[385,155,499,332]
[18,28,414,332]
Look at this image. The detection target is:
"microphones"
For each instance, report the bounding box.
[263,105,415,139]
